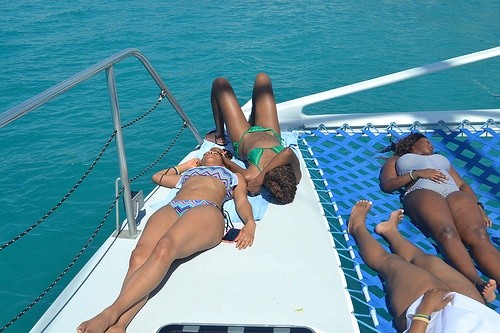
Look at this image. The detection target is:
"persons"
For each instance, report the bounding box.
[77,149,256,333]
[379,131,500,303]
[206,71,303,205]
[347,200,500,332]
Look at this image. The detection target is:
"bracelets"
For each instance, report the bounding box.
[173,166,180,175]
[409,169,417,181]
[413,313,431,320]
[413,316,431,323]
[476,201,484,209]
